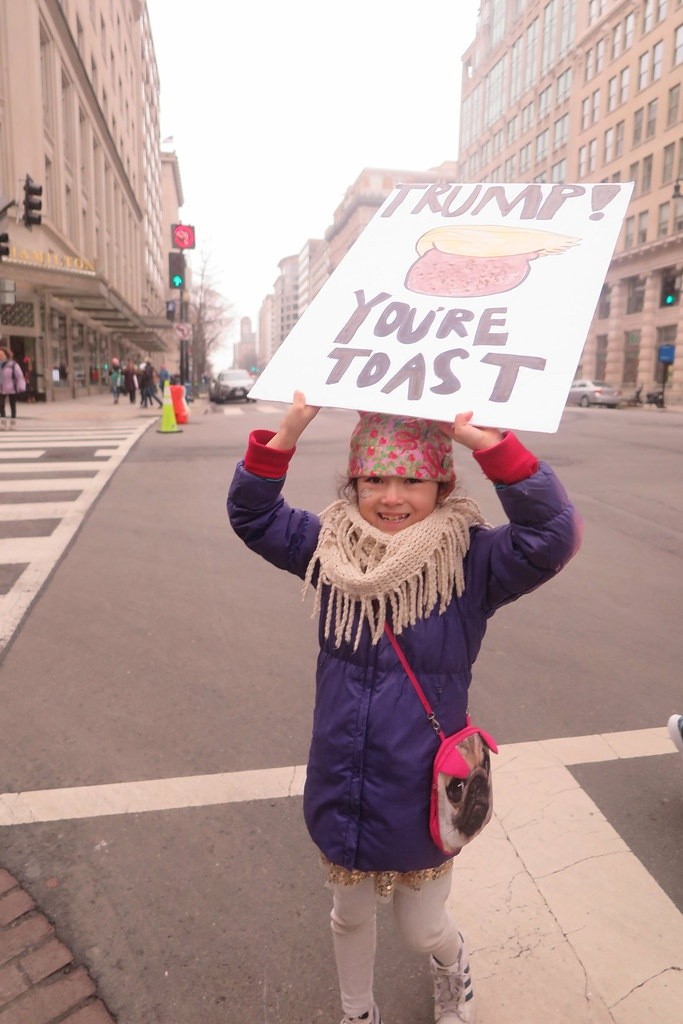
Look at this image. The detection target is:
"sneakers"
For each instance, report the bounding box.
[429,930,474,1024]
[339,1001,384,1024]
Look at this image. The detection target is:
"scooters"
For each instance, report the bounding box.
[627,383,664,408]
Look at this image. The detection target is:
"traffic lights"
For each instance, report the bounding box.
[168,253,185,288]
[0,232,9,255]
[23,174,42,231]
[659,276,675,309]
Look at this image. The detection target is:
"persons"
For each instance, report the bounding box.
[15,350,36,403]
[0,346,26,431]
[109,356,180,409]
[227,390,581,1024]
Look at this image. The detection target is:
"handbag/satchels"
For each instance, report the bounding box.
[429,725,500,857]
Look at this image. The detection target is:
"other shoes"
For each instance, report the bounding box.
[0,417,17,431]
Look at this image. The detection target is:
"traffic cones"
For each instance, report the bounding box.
[156,380,184,434]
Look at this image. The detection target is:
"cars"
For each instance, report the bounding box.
[567,379,623,409]
[208,376,217,401]
[213,370,257,404]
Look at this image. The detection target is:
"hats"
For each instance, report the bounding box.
[348,410,458,485]
